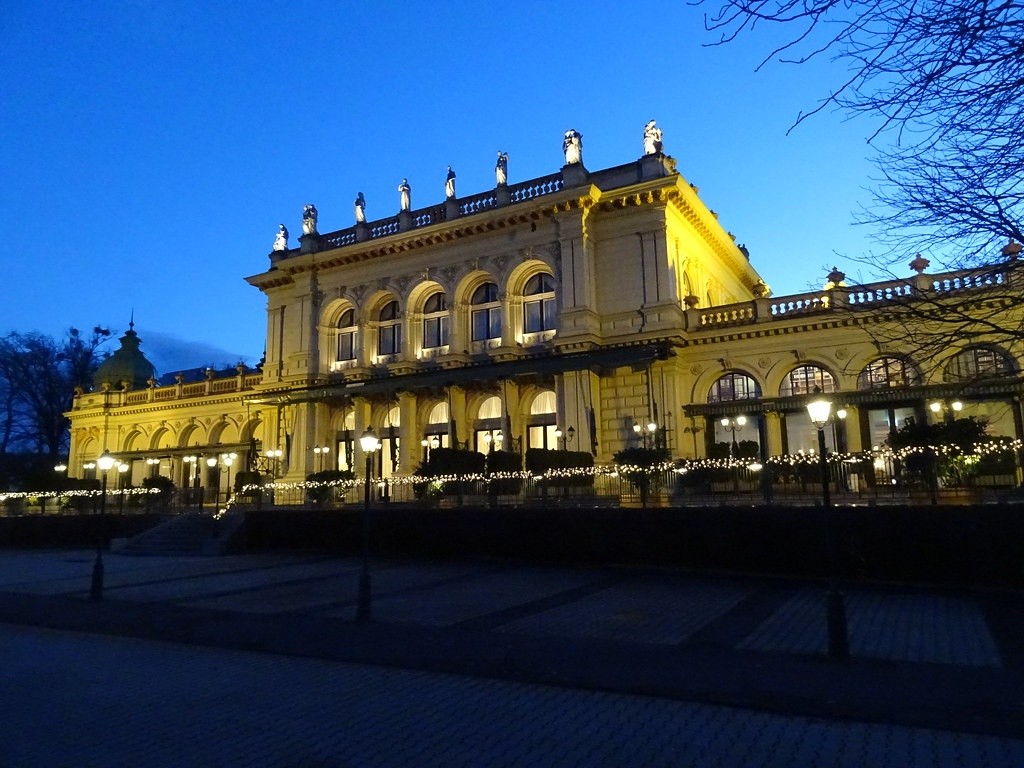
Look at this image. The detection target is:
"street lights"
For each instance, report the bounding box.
[222,453,237,502]
[722,412,747,460]
[359,425,379,504]
[207,456,232,517]
[805,385,835,506]
[98,449,116,516]
[117,463,129,515]
[314,443,330,473]
[54,461,67,495]
[555,425,575,450]
[633,420,657,449]
[147,458,160,476]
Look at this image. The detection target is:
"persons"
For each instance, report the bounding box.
[643,119,662,154]
[354,192,366,222]
[273,224,289,250]
[563,129,582,164]
[302,204,317,234]
[398,178,411,210]
[445,165,456,199]
[494,150,507,185]
